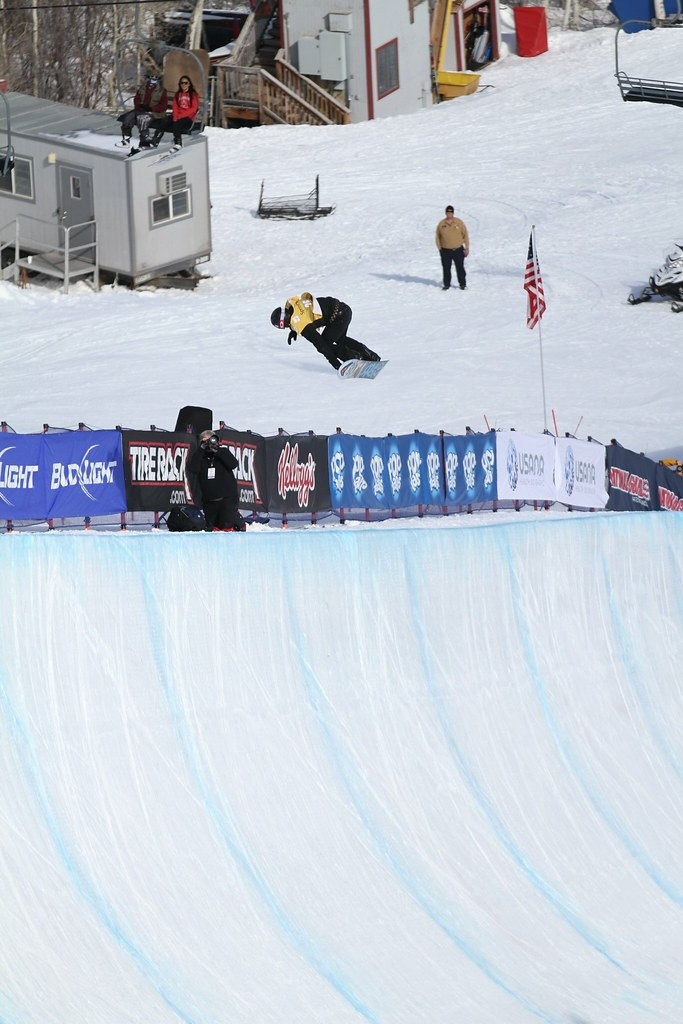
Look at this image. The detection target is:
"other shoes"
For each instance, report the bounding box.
[459,286,468,290]
[443,285,450,289]
[115,135,131,148]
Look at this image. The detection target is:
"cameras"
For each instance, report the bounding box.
[201,435,220,450]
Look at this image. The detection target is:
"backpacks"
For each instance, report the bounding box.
[167,504,206,532]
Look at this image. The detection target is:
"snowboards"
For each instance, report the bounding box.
[338,358,390,380]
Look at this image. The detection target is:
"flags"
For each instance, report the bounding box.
[524,232,546,329]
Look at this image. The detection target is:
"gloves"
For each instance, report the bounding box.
[287,330,298,345]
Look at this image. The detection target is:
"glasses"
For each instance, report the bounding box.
[181,82,189,85]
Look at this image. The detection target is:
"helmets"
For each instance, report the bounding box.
[270,306,290,329]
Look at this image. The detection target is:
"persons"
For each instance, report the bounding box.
[270,293,381,371]
[187,430,247,532]
[145,76,198,152]
[435,206,469,290]
[116,73,167,150]
